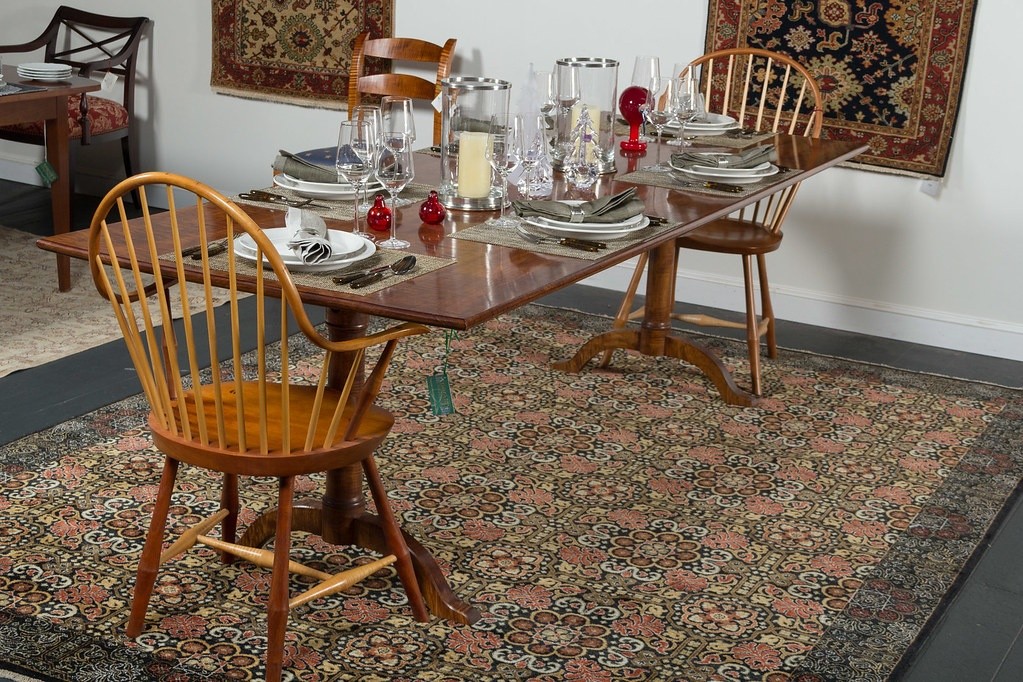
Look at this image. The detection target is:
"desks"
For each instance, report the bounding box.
[38,130,868,630]
[0,65,101,293]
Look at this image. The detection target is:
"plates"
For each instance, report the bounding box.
[668,153,779,184]
[239,228,364,261]
[0,63,73,90]
[520,200,649,240]
[272,173,386,201]
[232,237,376,272]
[664,112,740,135]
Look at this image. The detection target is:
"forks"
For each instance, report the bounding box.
[181,232,241,258]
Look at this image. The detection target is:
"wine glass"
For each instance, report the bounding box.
[485,65,579,229]
[631,55,700,172]
[335,95,415,249]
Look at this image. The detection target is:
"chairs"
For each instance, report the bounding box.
[87,169,450,682]
[555,51,824,407]
[0,7,150,220]
[343,30,461,149]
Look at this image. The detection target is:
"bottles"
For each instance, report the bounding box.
[418,223,445,253]
[418,191,446,225]
[367,194,392,230]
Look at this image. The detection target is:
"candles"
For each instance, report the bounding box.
[552,57,620,174]
[439,77,512,213]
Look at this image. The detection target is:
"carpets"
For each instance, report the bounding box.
[0,224,254,383]
[210,0,396,112]
[0,288,1023,682]
[699,0,980,183]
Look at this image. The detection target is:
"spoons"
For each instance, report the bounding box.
[350,256,417,287]
[237,191,314,207]
[516,231,598,252]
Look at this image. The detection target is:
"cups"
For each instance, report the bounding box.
[441,77,511,211]
[557,56,620,173]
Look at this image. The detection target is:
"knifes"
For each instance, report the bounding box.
[250,189,332,210]
[332,264,392,283]
